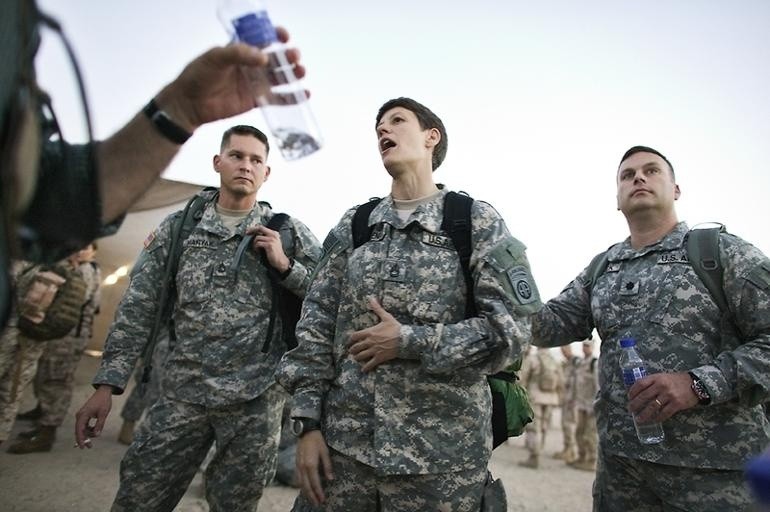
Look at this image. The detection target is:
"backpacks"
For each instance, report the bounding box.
[21,262,88,342]
[538,354,557,391]
[352,192,535,448]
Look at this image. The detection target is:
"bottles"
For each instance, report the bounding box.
[215,0,323,164]
[618,338,665,444]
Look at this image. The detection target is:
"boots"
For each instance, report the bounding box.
[6,402,56,454]
[519,454,537,469]
[118,420,135,445]
[553,445,596,471]
